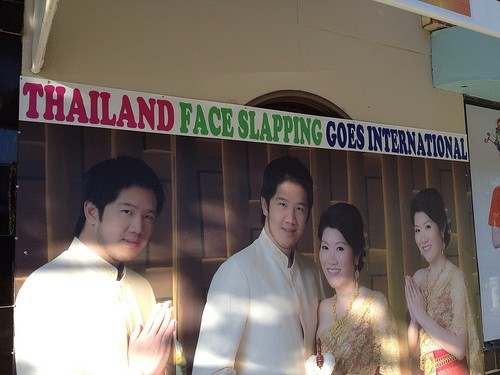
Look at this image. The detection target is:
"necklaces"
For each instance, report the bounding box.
[417,259,448,304]
[327,285,360,337]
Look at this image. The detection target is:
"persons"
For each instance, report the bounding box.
[12,156,176,375]
[406,188,484,375]
[193,157,400,375]
